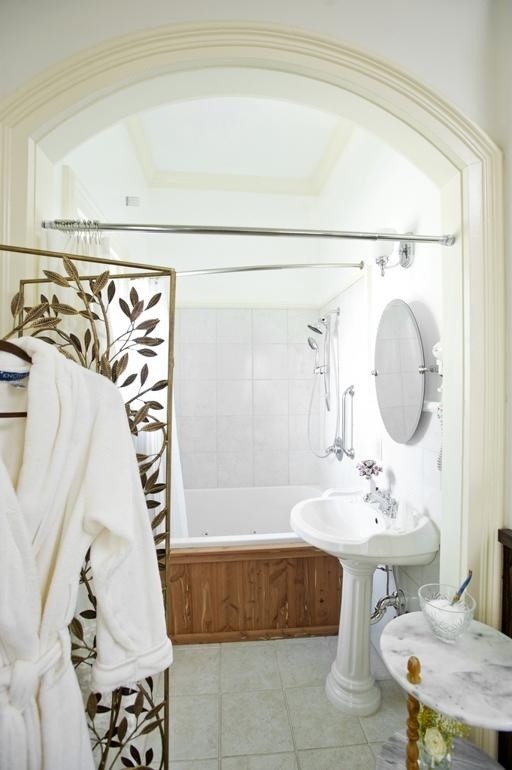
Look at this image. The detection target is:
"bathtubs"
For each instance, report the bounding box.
[155,485,325,546]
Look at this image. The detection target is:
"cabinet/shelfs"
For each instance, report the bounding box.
[377,611,512,770]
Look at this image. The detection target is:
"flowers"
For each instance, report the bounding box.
[413,705,465,763]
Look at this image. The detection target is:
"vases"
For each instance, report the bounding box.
[417,746,455,770]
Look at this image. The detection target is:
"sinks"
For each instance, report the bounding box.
[289,487,440,567]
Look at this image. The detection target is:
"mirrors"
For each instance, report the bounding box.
[375,297,422,445]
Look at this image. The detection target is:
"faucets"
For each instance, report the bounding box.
[364,490,398,519]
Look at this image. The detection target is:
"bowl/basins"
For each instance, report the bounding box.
[416,582,476,644]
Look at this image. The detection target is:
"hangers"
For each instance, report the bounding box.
[0,339,34,420]
